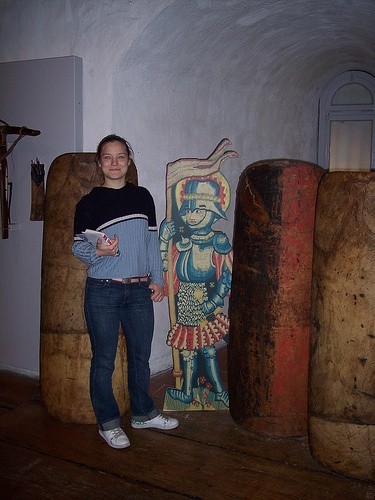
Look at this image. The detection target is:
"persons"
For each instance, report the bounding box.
[73,132,179,450]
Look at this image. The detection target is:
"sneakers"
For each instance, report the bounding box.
[99,426,130,449]
[130,413,179,430]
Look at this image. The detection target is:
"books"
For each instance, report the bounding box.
[82,229,121,257]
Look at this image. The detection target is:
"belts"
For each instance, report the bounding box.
[102,277,150,284]
